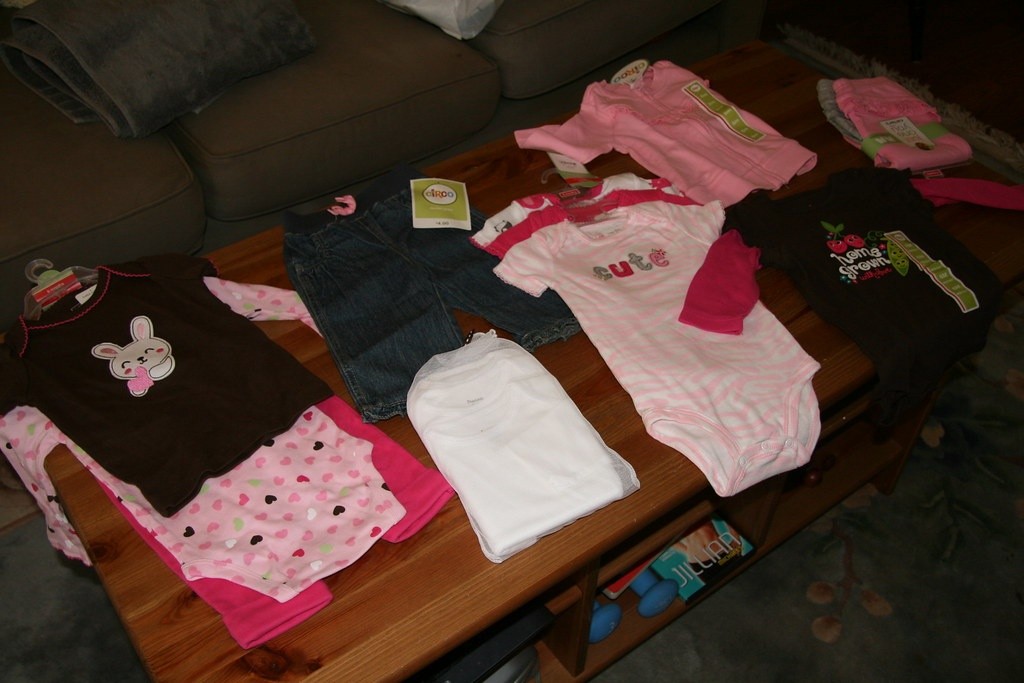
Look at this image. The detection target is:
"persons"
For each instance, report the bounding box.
[671,520,742,585]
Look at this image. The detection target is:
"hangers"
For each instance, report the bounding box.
[23,258,98,321]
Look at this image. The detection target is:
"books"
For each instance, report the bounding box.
[602,513,755,603]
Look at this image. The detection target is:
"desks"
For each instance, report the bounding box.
[0,40,1024,683]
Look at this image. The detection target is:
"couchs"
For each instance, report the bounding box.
[0,0,767,330]
